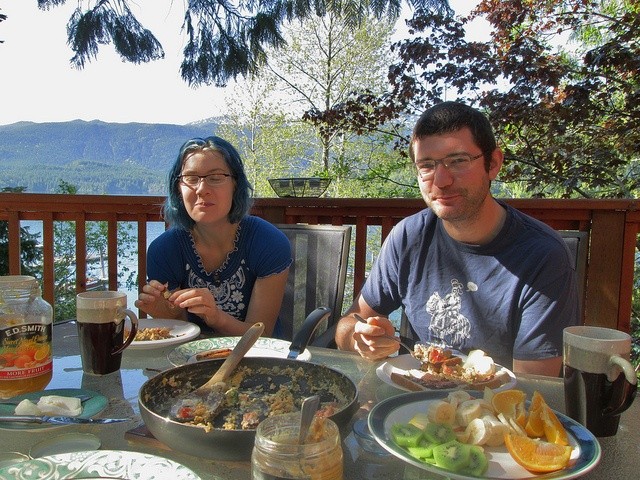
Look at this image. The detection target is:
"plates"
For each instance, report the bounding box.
[1,448,203,480]
[365,388,603,480]
[373,349,518,394]
[120,316,205,352]
[0,387,110,433]
[167,333,313,369]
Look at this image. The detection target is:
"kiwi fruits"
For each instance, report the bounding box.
[409,438,434,457]
[433,440,470,469]
[465,444,487,476]
[426,421,457,445]
[391,423,425,445]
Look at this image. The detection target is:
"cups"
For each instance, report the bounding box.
[560,323,640,439]
[74,290,140,378]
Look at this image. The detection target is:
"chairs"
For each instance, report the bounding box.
[271,218,352,347]
[555,229,591,325]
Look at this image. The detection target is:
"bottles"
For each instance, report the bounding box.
[249,410,346,479]
[0,272,48,400]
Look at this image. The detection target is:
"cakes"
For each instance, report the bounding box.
[413,343,461,369]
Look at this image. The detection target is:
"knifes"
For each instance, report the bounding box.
[0,413,135,427]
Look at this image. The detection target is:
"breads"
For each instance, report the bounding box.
[391,369,468,389]
[439,363,511,388]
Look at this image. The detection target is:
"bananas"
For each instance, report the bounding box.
[509,417,526,436]
[411,389,504,446]
[499,413,515,434]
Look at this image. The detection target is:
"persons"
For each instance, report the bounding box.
[133,136,294,338]
[335,101,580,378]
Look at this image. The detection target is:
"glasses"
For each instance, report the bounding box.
[177,173,236,186]
[414,151,484,176]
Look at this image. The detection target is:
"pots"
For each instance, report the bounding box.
[136,304,362,448]
